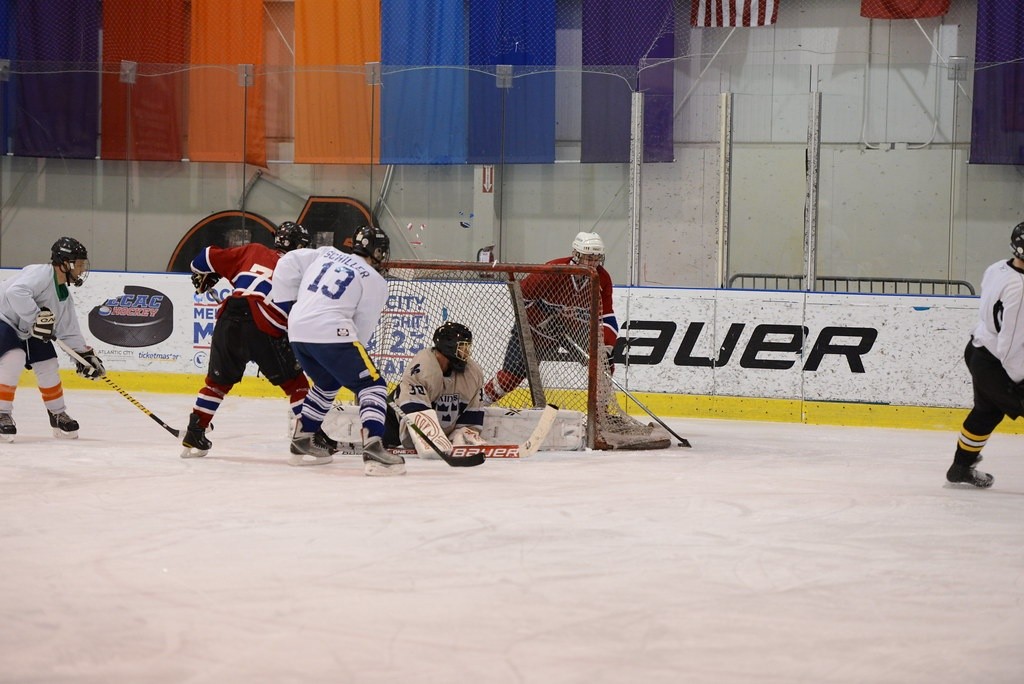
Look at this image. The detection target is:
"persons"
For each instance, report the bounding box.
[481,232,620,425]
[181,221,333,466]
[381,321,487,459]
[0,236,106,443]
[943,222,1024,489]
[270,224,407,477]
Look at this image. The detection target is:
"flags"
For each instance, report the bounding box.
[860,0,951,20]
[691,0,779,27]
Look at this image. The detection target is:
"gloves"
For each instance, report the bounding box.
[603,344,615,377]
[75,346,106,381]
[539,315,569,343]
[190,272,220,295]
[30,307,54,344]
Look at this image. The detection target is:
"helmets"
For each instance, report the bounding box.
[273,222,310,251]
[433,323,472,373]
[351,224,390,276]
[51,237,90,287]
[1009,222,1024,260]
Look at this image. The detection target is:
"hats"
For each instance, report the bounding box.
[572,231,605,273]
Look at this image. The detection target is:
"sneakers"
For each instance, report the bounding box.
[47,409,80,439]
[943,453,995,489]
[0,410,17,443]
[179,414,212,459]
[361,427,407,477]
[288,418,334,465]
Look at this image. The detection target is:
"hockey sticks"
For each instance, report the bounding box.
[558,329,694,448]
[387,395,487,467]
[209,289,365,457]
[385,402,559,452]
[53,334,215,439]
